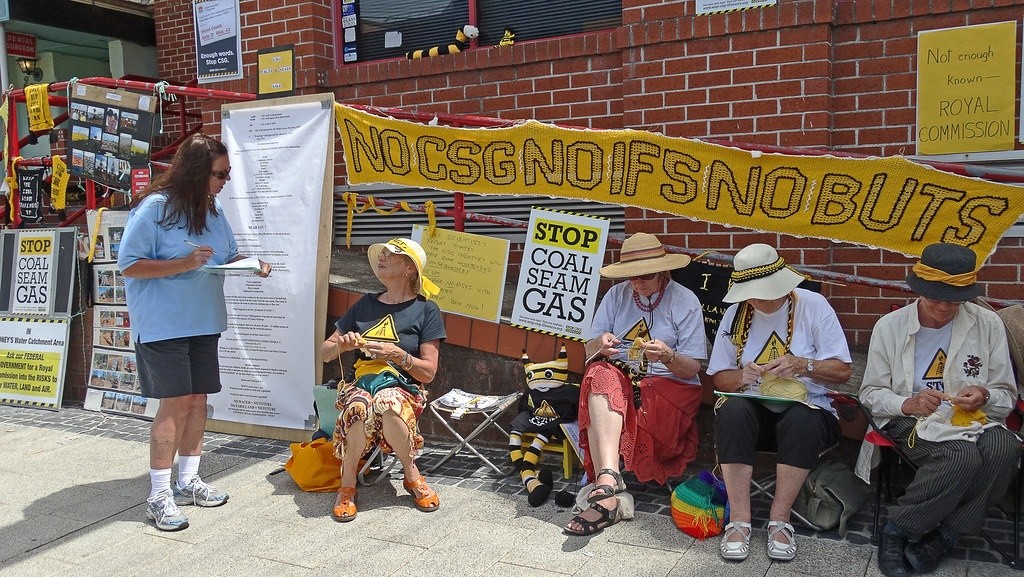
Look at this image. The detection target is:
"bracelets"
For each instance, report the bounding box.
[400,353,413,371]
[664,352,675,365]
[985,393,990,404]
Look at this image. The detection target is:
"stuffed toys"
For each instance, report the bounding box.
[508,342,580,507]
[406,25,479,59]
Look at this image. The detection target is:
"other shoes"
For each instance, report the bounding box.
[720,522,751,560]
[767,520,797,560]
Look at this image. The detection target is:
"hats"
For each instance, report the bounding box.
[722,244,806,303]
[906,242,982,301]
[598,230,691,277]
[368,238,440,300]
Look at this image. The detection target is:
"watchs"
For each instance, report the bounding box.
[806,359,813,373]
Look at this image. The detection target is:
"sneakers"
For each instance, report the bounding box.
[878,521,913,577]
[174,473,229,507]
[145,489,189,531]
[905,527,953,573]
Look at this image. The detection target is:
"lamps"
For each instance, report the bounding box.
[15,57,43,81]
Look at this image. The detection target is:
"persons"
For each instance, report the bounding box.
[705,243,853,560]
[117,133,273,530]
[563,232,708,536]
[854,243,1024,577]
[320,238,447,521]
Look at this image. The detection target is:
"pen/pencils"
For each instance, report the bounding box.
[184,240,217,254]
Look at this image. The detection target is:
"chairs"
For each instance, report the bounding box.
[521,381,581,479]
[846,395,1021,569]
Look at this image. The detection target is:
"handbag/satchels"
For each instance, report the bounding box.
[670,470,730,542]
[285,438,369,493]
[801,459,863,536]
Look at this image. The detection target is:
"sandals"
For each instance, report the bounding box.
[333,487,358,523]
[402,475,439,512]
[586,468,627,503]
[563,500,622,536]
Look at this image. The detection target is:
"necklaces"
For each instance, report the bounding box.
[633,283,665,312]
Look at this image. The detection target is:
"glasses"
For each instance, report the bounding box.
[925,296,966,304]
[626,273,656,281]
[209,166,231,180]
[377,250,414,264]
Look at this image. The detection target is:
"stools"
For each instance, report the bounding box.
[749,434,840,531]
[429,388,523,477]
